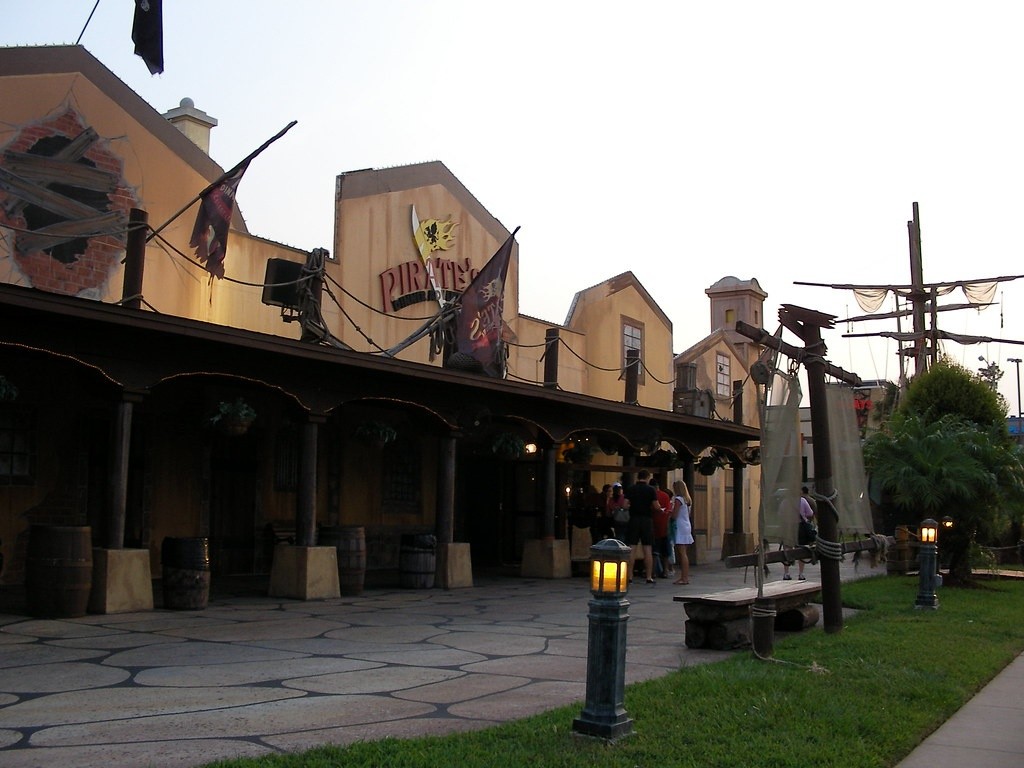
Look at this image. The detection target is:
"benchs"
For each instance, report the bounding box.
[672,581,825,651]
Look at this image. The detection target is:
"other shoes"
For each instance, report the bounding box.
[798,576,806,580]
[646,579,656,584]
[628,578,632,585]
[783,575,792,580]
[672,579,690,584]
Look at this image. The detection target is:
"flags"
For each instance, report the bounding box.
[189,162,250,281]
[455,234,514,363]
[132,0,164,74]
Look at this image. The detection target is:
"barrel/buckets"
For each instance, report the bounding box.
[162,536,212,610]
[319,524,367,595]
[23,522,92,616]
[399,532,438,588]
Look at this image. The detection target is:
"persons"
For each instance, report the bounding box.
[777,486,818,580]
[595,469,695,585]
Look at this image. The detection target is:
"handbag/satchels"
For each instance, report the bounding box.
[614,509,630,522]
[801,522,818,542]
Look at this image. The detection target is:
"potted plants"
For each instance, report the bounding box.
[562,441,601,465]
[489,431,525,461]
[353,417,399,449]
[698,456,718,475]
[200,399,258,436]
